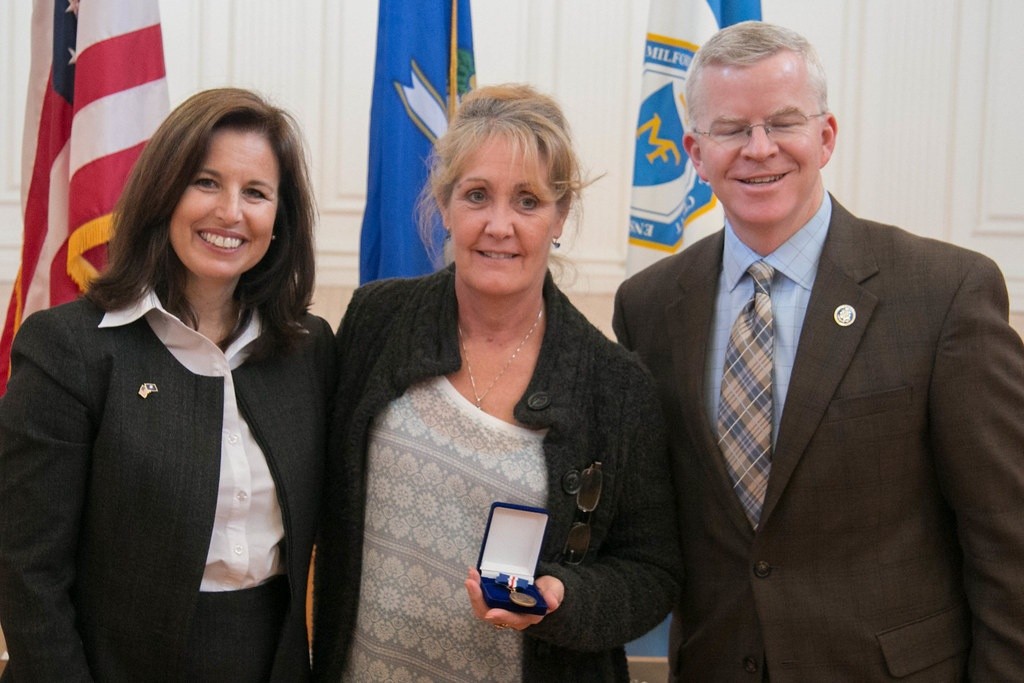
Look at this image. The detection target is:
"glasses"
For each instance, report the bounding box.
[560,461,604,567]
[691,110,825,144]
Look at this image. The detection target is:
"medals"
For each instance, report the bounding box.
[510,591,538,608]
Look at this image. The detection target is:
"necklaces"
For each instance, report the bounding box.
[458,296,545,410]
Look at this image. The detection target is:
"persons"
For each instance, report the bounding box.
[612,21,1024,683]
[309,83,679,683]
[0,87,341,683]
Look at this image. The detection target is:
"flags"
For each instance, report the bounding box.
[0,0,175,399]
[358,1,480,289]
[625,1,762,276]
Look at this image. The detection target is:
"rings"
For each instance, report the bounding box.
[493,623,509,632]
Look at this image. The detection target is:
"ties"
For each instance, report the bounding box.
[715,258,777,534]
